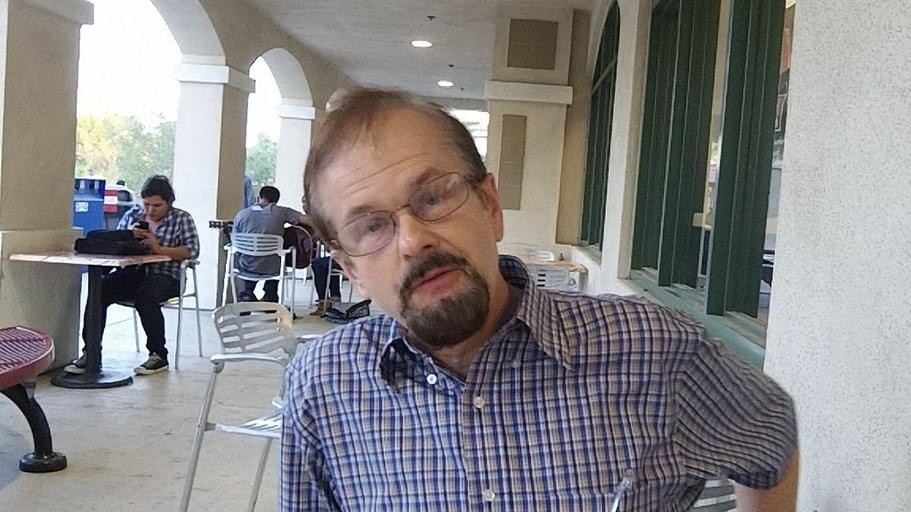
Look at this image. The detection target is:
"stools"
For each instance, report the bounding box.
[0,325,67,472]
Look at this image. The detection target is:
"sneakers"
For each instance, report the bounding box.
[64,344,104,375]
[134,350,169,377]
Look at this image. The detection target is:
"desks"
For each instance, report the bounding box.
[524,259,587,274]
[11,250,174,389]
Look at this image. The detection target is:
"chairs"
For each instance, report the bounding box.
[292,224,321,285]
[526,262,570,292]
[322,256,352,317]
[117,259,201,370]
[182,302,325,512]
[221,232,297,323]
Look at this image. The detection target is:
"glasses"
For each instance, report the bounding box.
[328,171,476,257]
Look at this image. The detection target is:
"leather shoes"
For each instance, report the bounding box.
[309,298,341,315]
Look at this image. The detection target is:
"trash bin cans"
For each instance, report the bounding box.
[72,177,106,273]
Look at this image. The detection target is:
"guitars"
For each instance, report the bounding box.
[209,221,316,268]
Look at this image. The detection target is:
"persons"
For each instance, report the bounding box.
[63,175,201,377]
[244,177,256,209]
[310,256,343,316]
[232,185,311,316]
[276,86,800,512]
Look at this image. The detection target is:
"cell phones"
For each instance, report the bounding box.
[136,222,149,229]
